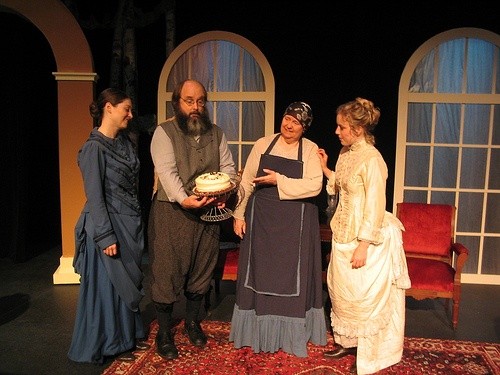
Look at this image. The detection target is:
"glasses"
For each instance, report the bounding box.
[180,96,206,106]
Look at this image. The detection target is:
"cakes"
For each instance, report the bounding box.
[194,172,231,192]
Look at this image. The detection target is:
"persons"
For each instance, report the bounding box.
[229,102,328,357]
[148,80,236,359]
[318,97,412,375]
[67,87,150,363]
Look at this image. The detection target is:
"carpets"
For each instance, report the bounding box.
[103,319,500,375]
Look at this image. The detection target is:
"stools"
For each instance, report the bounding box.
[203,203,238,311]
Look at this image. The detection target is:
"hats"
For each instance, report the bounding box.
[283,102,313,131]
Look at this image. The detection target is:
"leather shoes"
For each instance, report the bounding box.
[117,351,134,362]
[155,331,178,359]
[349,360,357,373]
[183,321,207,347]
[134,341,152,350]
[323,346,354,358]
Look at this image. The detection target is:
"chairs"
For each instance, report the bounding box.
[396,203,469,331]
